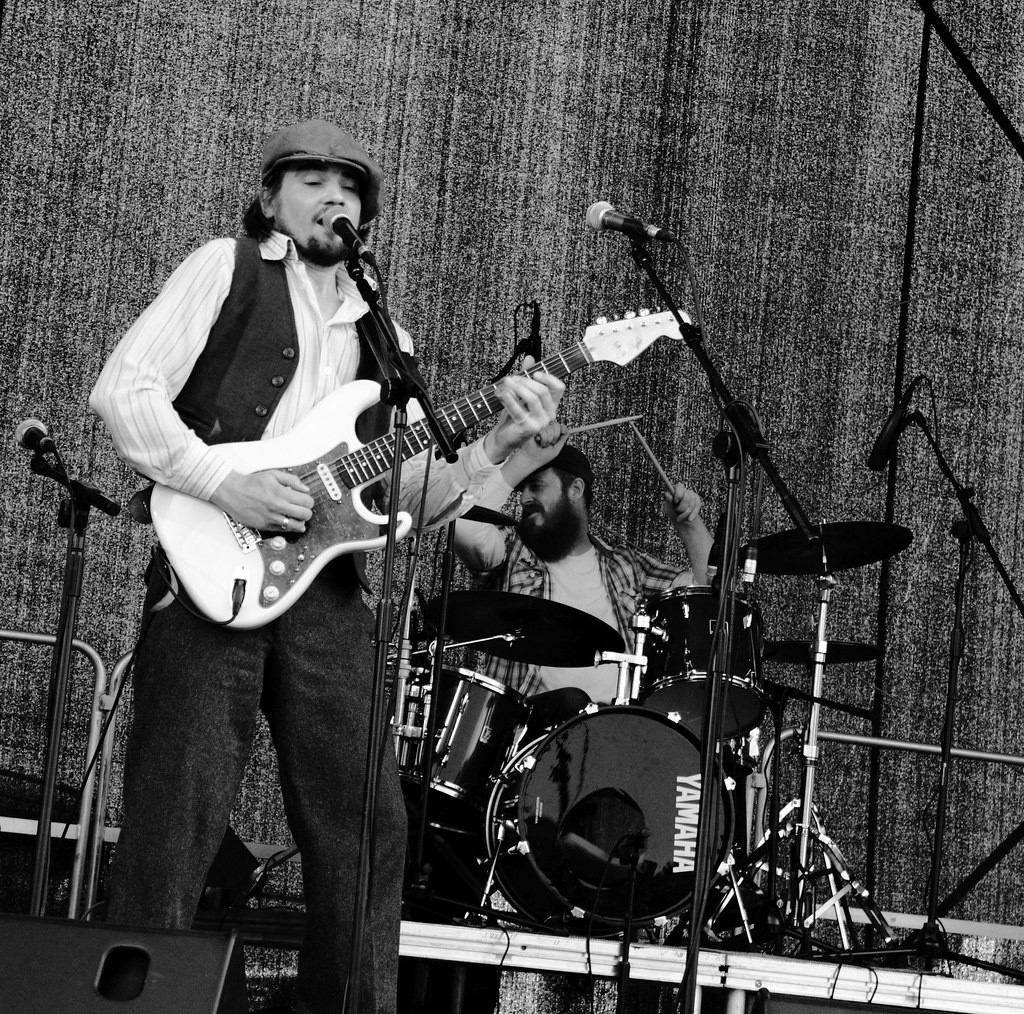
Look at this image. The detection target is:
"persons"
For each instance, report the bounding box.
[449,415,715,747]
[88,117,568,1014]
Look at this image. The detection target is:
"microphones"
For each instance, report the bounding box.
[324,205,375,266]
[867,381,919,471]
[15,420,56,452]
[618,830,651,866]
[585,201,678,242]
[525,303,541,365]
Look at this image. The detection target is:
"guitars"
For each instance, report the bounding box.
[147,304,695,631]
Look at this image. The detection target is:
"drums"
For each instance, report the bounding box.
[394,664,533,835]
[639,583,768,742]
[485,704,737,938]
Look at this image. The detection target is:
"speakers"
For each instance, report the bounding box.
[0,912,249,1014]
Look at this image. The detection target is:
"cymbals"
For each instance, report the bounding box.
[423,590,626,669]
[736,521,914,576]
[771,637,882,666]
[460,503,520,527]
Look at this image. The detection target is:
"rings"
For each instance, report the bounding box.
[281,518,289,529]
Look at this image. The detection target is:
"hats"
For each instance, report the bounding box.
[260,118,385,225]
[512,444,595,493]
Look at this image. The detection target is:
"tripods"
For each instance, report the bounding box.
[401,518,571,941]
[666,410,1024,980]
